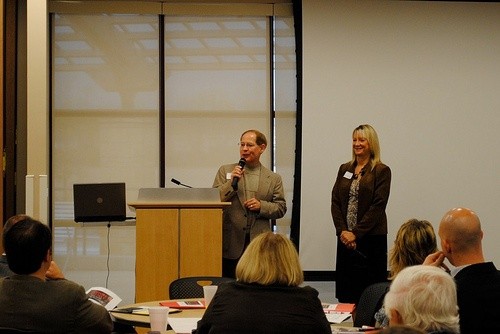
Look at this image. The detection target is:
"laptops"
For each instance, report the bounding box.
[72,182,135,222]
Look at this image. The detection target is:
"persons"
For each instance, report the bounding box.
[421,207,500,334]
[0,217,114,334]
[212,129,288,279]
[0,214,66,281]
[388,219,437,281]
[382,264,459,334]
[330,124,393,304]
[190,230,333,334]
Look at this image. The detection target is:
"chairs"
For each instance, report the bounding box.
[192,311,328,334]
[2,313,117,333]
[355,278,395,329]
[107,295,355,326]
[167,276,237,299]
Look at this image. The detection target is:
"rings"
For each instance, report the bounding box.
[250,205,253,208]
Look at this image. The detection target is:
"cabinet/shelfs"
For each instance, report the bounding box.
[128,202,231,303]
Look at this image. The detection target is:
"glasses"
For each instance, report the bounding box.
[238,142,259,149]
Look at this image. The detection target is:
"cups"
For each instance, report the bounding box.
[203,284,218,309]
[147,306,173,334]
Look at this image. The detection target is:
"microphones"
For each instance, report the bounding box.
[171,178,192,188]
[231,158,246,189]
